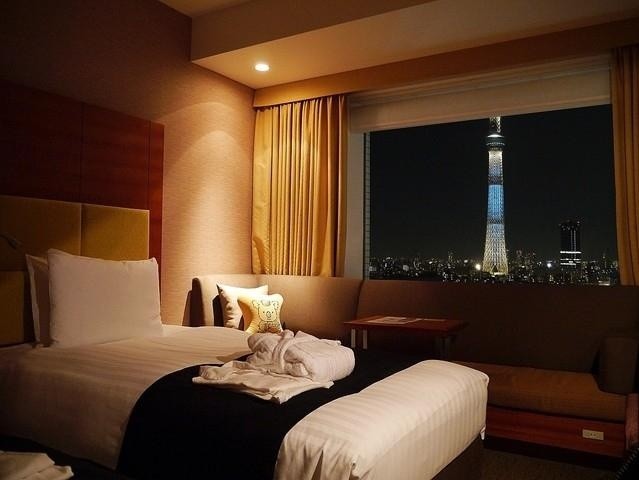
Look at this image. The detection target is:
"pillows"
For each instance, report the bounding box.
[24,253,51,349]
[216,282,268,330]
[237,293,284,334]
[47,247,163,350]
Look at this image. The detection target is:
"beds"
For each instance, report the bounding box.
[0,195,489,480]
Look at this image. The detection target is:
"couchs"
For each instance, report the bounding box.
[192,274,639,457]
